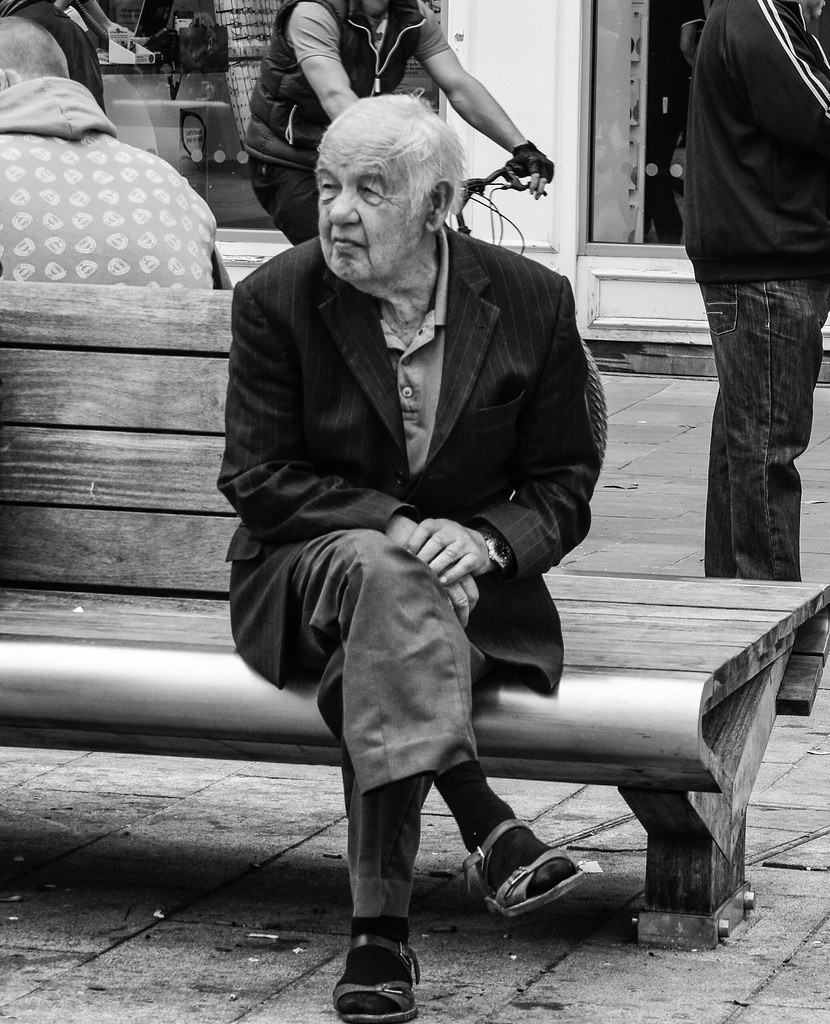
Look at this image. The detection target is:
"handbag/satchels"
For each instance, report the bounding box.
[180,12,229,73]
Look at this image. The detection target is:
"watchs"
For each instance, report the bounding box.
[474,527,513,575]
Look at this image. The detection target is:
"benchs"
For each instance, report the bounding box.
[0,280,830,952]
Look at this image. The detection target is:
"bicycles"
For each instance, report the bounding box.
[450,159,547,255]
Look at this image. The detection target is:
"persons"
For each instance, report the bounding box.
[643,0,708,244]
[0,0,554,290]
[684,0,830,581]
[217,88,600,1024]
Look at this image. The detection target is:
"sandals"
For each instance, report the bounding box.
[464,819,585,917]
[332,935,420,1023]
